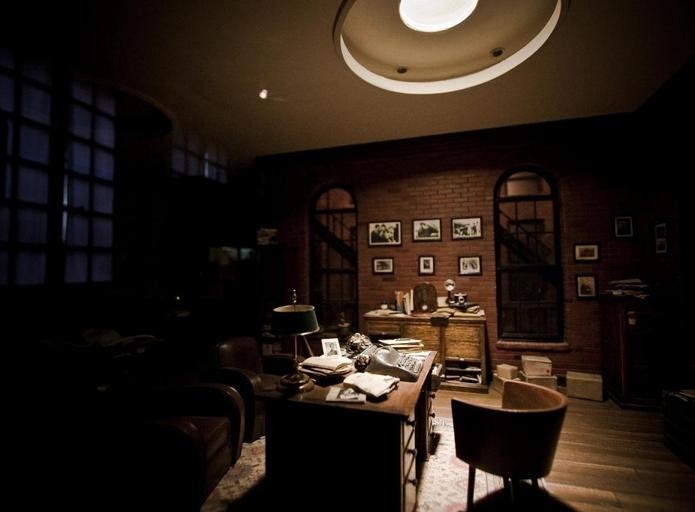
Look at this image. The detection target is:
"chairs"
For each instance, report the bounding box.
[451,383,567,511]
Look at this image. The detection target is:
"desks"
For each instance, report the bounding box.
[259,349,444,512]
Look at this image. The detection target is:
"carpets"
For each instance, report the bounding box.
[201,409,548,512]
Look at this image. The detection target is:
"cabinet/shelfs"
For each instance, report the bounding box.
[361,308,486,392]
[604,287,666,408]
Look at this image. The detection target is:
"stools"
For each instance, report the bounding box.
[661,386,695,472]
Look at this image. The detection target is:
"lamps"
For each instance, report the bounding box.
[267,298,325,389]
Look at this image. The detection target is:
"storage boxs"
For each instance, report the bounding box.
[520,370,558,389]
[567,370,605,400]
[496,362,519,380]
[521,354,552,375]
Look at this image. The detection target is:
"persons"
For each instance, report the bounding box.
[371,224,393,242]
[328,343,337,356]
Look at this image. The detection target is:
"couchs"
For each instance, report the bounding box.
[70,332,243,505]
[170,312,300,445]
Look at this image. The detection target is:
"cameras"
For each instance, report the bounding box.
[453,293,468,304]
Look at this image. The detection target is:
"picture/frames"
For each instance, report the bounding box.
[574,214,669,300]
[418,255,435,276]
[411,217,443,242]
[451,216,483,241]
[459,256,482,276]
[367,220,403,247]
[372,256,394,274]
